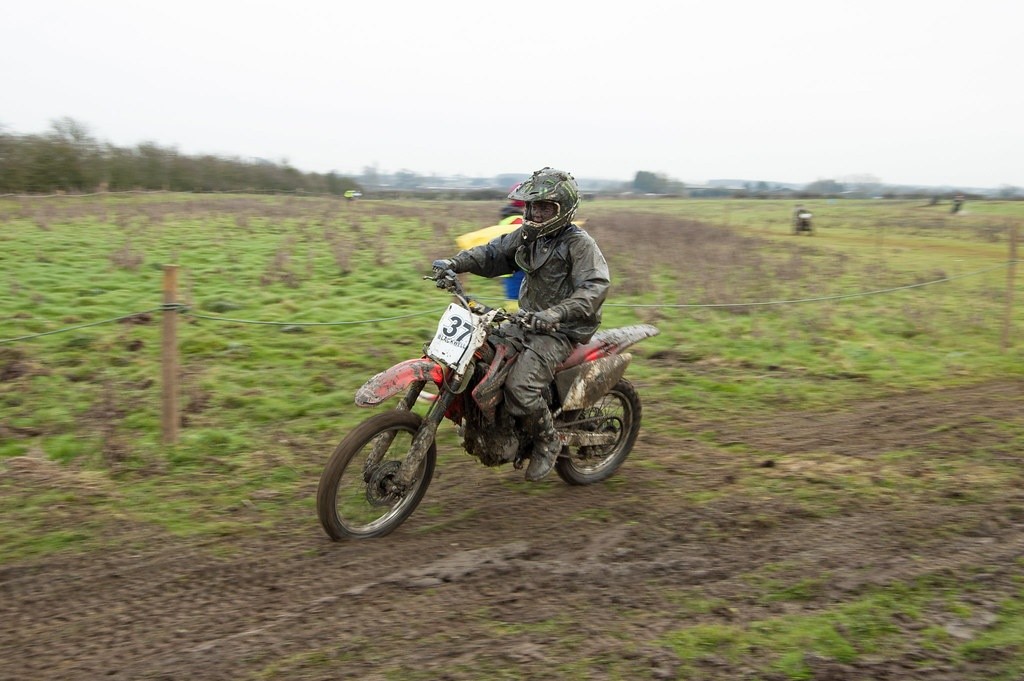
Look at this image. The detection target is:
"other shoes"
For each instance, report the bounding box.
[524,429,561,480]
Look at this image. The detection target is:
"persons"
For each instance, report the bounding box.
[951,195,964,214]
[794,204,813,233]
[431,167,610,482]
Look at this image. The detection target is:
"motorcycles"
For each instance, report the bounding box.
[315,268,661,542]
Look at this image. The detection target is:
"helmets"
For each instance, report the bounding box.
[508,166,580,242]
